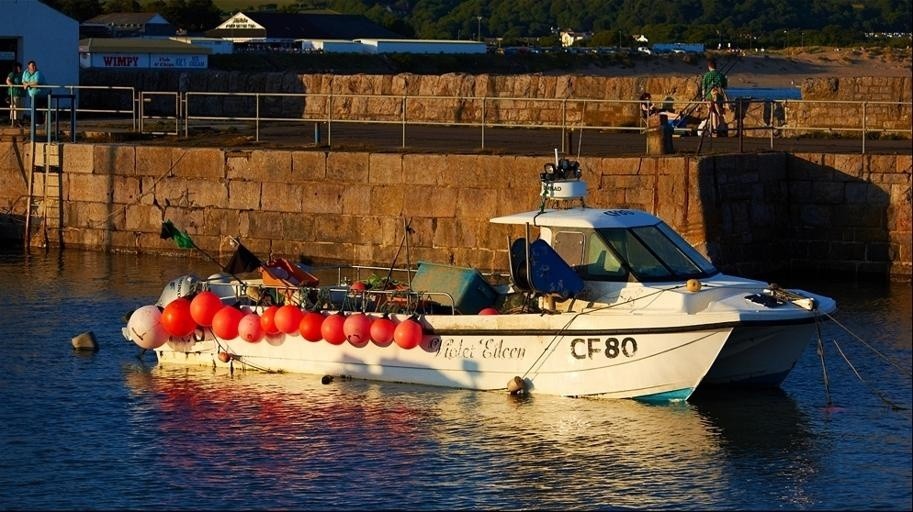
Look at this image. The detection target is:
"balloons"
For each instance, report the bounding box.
[352,281,366,289]
[477,307,499,314]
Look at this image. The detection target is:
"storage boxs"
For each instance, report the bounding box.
[409,260,501,316]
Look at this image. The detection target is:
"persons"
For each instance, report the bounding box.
[701,60,728,138]
[22,61,52,127]
[639,92,681,119]
[6,62,27,124]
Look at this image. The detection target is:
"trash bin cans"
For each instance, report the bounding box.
[646,114,673,156]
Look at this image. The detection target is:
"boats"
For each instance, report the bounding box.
[121,148,839,402]
